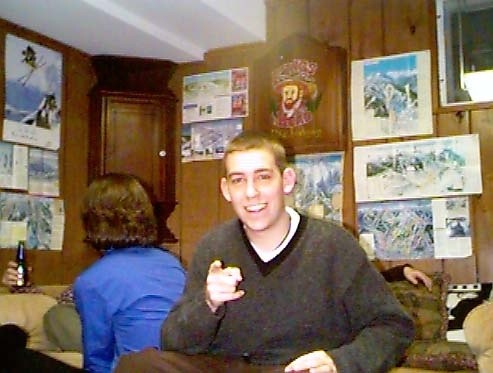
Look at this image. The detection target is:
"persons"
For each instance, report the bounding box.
[1,260,73,302]
[72,172,188,373]
[380,263,437,292]
[160,128,416,373]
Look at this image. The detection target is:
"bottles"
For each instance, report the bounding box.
[16,240,28,289]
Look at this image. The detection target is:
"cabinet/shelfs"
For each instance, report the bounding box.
[89,54,179,244]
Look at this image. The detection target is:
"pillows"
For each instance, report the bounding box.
[389,271,481,373]
